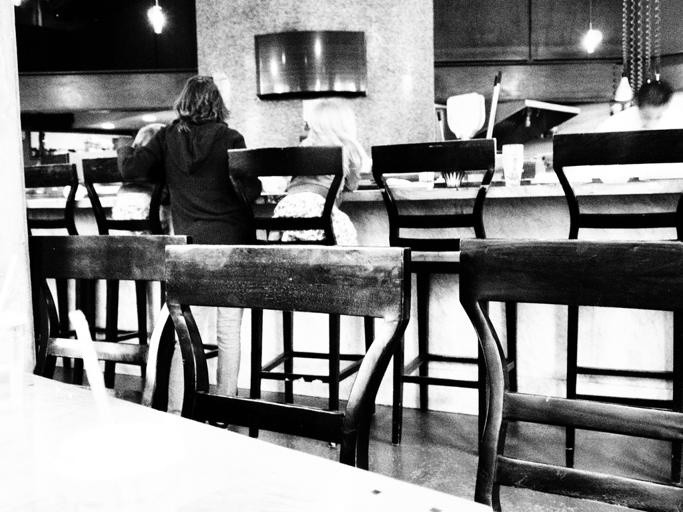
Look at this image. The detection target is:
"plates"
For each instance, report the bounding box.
[389,184,432,191]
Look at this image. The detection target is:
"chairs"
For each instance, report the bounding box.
[371,138,517,456]
[459,238,683,511]
[24,164,97,386]
[29,234,192,418]
[553,129,683,481]
[164,244,412,471]
[82,157,170,391]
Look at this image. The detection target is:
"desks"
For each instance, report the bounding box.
[25,175,682,420]
[24,373,492,511]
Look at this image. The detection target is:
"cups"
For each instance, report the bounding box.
[502,144,524,186]
[418,172,434,188]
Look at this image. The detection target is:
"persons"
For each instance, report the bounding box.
[113,123,177,345]
[267,97,361,246]
[117,76,263,429]
